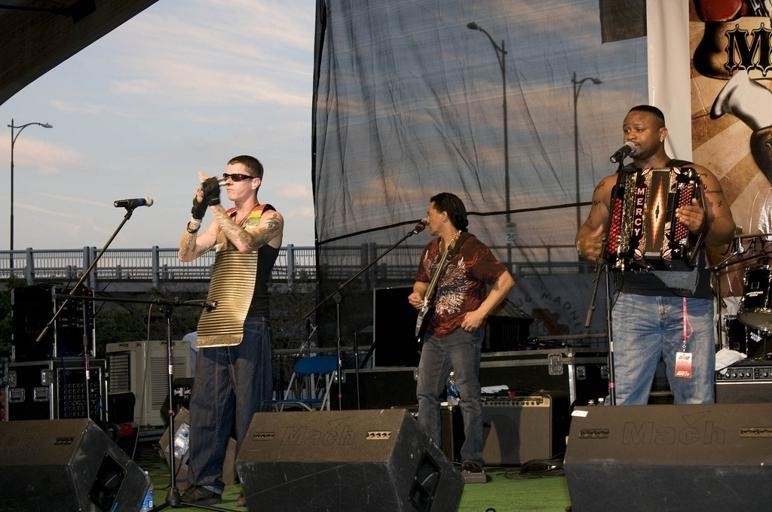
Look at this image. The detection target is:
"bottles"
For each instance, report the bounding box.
[445,372,459,406]
[139,471,154,512]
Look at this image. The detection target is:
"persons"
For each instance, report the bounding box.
[407,192,516,470]
[574,105,737,408]
[174,155,283,508]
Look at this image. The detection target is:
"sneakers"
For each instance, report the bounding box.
[171,486,222,508]
[463,462,482,473]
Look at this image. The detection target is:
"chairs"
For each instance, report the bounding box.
[263,354,338,413]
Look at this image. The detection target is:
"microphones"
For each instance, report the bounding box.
[113,196,154,210]
[206,300,218,314]
[610,140,639,166]
[414,217,429,233]
[712,287,727,309]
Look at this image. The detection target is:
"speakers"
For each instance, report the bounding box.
[713,366,772,405]
[561,404,772,511]
[235,406,464,512]
[470,393,552,469]
[372,285,431,367]
[341,367,421,410]
[0,417,152,512]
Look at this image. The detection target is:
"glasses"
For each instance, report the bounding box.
[223,173,254,181]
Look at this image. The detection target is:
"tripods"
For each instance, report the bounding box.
[52,292,241,512]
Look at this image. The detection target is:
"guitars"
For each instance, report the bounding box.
[411,230,463,351]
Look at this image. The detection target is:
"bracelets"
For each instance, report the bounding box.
[187,221,201,233]
[576,238,584,259]
